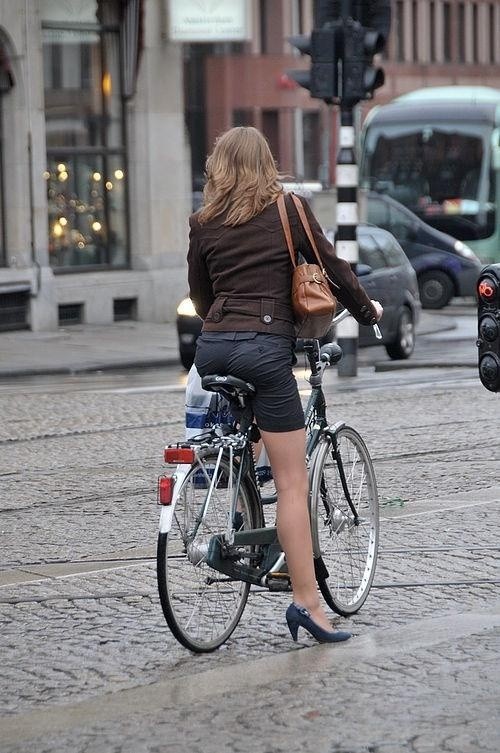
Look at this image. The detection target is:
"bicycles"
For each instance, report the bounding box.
[156,305,382,652]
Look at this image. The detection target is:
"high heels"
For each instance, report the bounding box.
[286,603,351,643]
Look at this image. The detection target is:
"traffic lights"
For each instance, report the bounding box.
[343,20,385,100]
[473,262,500,392]
[284,19,339,99]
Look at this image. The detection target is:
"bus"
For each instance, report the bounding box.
[357,83,500,297]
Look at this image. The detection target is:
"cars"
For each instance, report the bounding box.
[175,223,422,370]
[365,191,485,308]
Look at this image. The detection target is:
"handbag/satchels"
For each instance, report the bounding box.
[290,264,337,338]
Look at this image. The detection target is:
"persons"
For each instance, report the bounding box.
[187,126,385,639]
